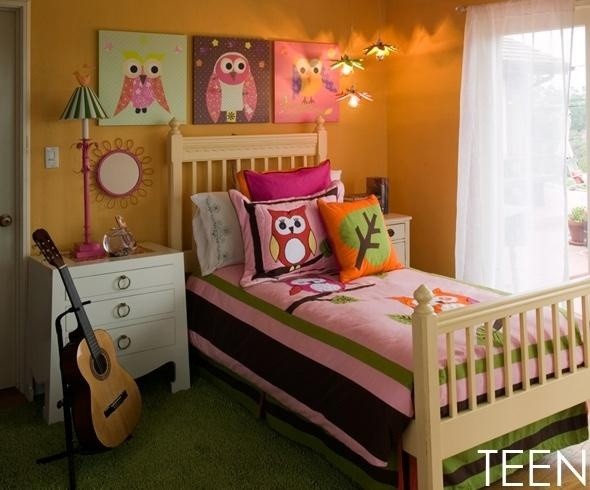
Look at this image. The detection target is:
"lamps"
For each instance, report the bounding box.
[330,51,365,75]
[58,85,107,262]
[334,85,374,110]
[363,38,401,62]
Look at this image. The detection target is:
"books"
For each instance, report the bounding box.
[345,175,389,215]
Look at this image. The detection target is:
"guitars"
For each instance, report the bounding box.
[30,228,142,453]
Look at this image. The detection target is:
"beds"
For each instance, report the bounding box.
[168,114,590,490]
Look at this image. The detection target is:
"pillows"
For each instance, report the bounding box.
[188,159,407,288]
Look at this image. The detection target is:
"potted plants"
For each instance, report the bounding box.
[567,207,588,245]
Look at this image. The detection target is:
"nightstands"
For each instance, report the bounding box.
[26,241,195,433]
[382,214,411,271]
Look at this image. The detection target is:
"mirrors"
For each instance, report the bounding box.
[85,137,153,208]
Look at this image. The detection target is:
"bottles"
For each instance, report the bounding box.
[103,227,131,257]
